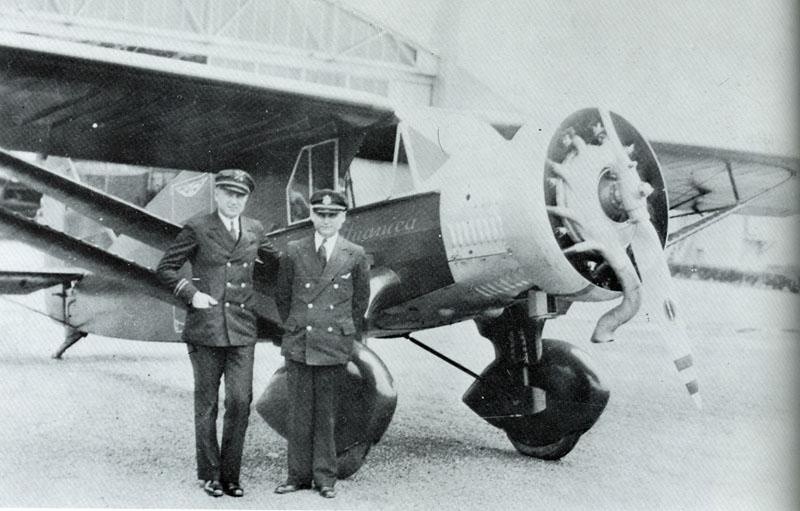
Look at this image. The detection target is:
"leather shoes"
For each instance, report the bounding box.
[226,482,244,497]
[274,480,313,495]
[205,476,225,498]
[315,481,339,499]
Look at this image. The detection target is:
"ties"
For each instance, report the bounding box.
[228,221,237,242]
[317,238,328,270]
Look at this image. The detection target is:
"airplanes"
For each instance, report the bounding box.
[0,29,800,479]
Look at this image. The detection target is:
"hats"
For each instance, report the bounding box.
[213,169,255,196]
[310,189,348,215]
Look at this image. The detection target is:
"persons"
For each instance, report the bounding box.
[156,169,282,496]
[275,189,369,498]
